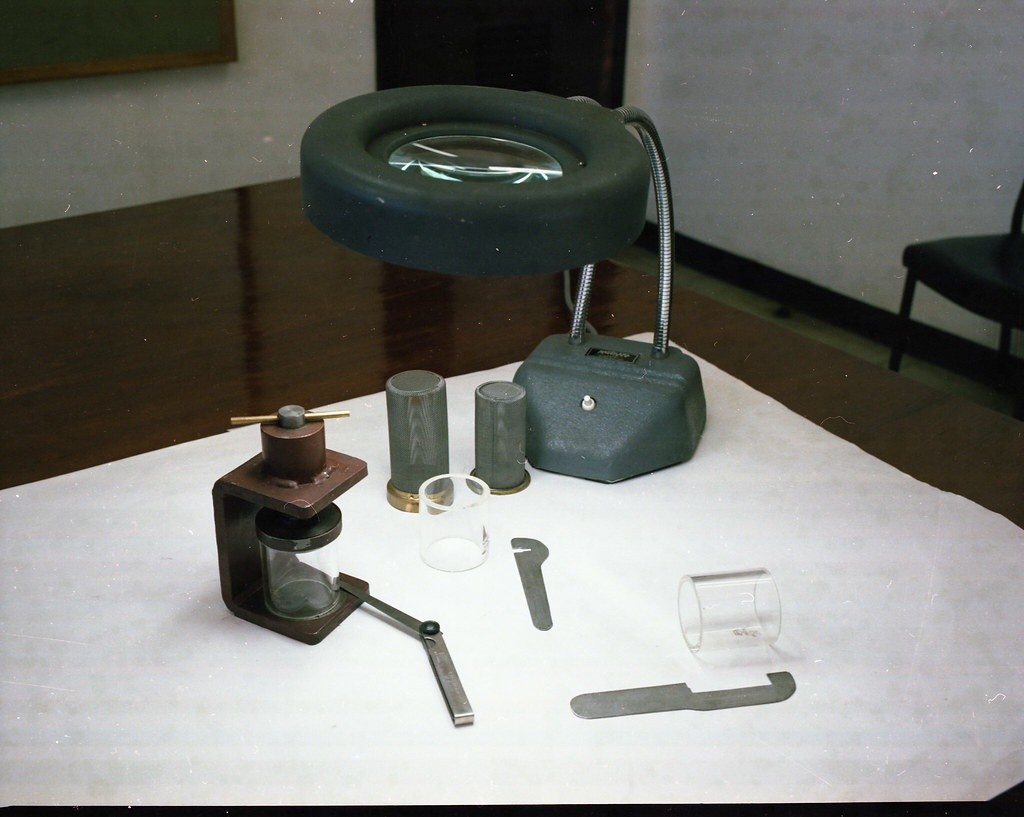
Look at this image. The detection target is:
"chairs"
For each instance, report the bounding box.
[888,186,1024,420]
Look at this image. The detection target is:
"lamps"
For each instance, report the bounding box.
[299,84,706,483]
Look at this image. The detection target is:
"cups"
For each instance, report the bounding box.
[255,502,343,619]
[418,473,490,569]
[680,569,782,652]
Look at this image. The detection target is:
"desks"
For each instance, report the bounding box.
[0,175,1024,817]
